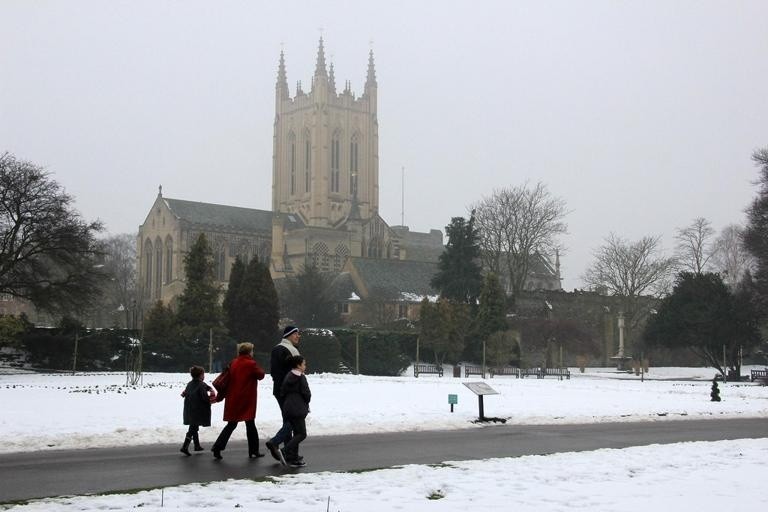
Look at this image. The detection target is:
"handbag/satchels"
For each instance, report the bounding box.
[213,369,229,398]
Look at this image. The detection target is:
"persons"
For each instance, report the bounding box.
[264,326,306,464]
[275,352,312,468]
[210,342,266,461]
[176,364,214,457]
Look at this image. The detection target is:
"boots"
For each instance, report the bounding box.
[193,436,203,451]
[181,438,192,456]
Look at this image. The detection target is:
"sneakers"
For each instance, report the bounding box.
[266,442,306,468]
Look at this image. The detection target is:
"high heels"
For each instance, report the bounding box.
[249,452,264,458]
[212,446,223,459]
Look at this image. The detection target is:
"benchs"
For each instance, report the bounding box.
[413,362,570,380]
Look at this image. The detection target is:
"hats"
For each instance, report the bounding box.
[283,326,298,338]
